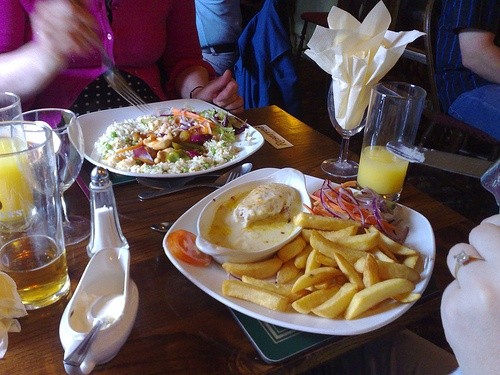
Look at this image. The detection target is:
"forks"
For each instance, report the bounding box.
[105,69,153,115]
[136,172,222,189]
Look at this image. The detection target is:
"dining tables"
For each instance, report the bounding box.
[0,104,477,375]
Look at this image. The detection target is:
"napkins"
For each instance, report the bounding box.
[0,273,29,359]
[304,0,427,130]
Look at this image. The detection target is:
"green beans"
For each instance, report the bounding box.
[104,132,210,174]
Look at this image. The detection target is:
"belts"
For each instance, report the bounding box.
[202,44,237,53]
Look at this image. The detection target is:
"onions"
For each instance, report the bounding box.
[318,179,409,245]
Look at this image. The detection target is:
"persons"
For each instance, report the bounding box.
[194,0,242,76]
[440,211,500,375]
[0,0,245,134]
[435,0,500,140]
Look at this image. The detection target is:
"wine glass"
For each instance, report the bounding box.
[10,107,92,247]
[320,79,376,178]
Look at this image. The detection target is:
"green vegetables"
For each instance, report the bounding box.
[201,108,235,143]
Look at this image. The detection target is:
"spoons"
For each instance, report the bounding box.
[136,163,252,200]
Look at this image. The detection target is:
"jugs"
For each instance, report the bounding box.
[59,247,138,374]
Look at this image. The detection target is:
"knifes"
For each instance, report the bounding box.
[386,140,494,179]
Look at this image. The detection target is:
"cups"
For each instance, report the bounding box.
[0,91,22,123]
[0,122,71,311]
[356,82,426,202]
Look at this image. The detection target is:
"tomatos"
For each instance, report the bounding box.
[167,228,212,265]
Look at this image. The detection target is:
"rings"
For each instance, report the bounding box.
[454,251,484,276]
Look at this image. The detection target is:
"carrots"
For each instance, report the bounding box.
[304,181,397,236]
[170,105,216,128]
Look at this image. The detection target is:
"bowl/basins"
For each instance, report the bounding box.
[197,168,313,266]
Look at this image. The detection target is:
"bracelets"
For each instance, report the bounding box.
[190,86,203,99]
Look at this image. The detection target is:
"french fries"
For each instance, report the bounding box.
[222,212,423,320]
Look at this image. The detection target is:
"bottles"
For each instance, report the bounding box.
[86,166,131,256]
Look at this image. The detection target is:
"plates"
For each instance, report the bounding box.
[67,98,264,178]
[162,168,435,335]
